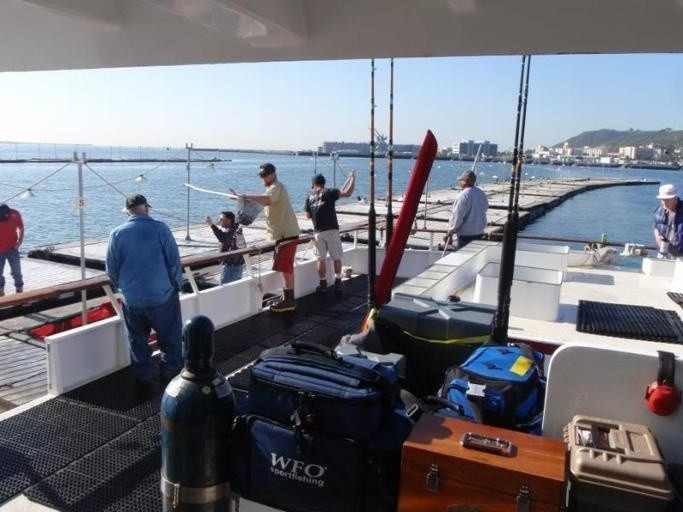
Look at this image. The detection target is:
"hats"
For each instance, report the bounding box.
[0,204,11,222]
[457,169,476,183]
[256,163,276,176]
[654,181,679,200]
[126,193,152,210]
[311,173,325,186]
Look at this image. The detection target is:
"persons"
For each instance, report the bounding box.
[230,163,300,312]
[204,211,244,285]
[303,173,355,295]
[653,184,683,259]
[444,170,488,249]
[0,204,25,295]
[106,194,185,395]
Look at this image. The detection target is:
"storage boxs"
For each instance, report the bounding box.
[565,414,675,512]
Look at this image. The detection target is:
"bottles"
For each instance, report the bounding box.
[158,315,236,512]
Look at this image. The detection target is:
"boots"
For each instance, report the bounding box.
[270,288,298,313]
[330,277,342,291]
[316,278,328,293]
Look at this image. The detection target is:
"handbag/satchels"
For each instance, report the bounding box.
[438,342,544,435]
[232,338,410,511]
